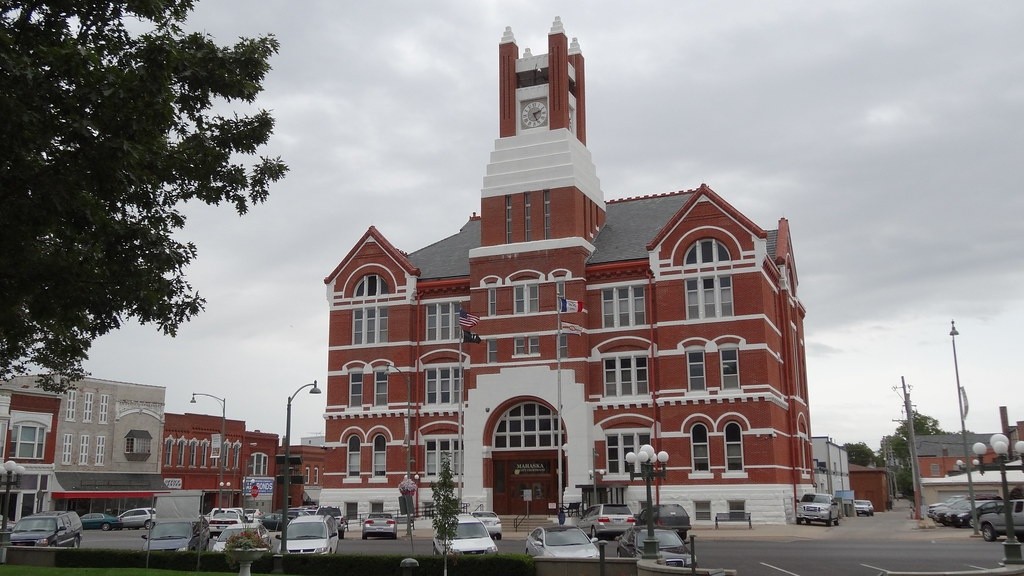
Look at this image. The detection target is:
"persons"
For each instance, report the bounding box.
[558,508,565,526]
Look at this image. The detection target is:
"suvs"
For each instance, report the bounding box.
[141,517,211,552]
[432,511,503,555]
[579,503,691,541]
[10,510,83,548]
[245,509,262,518]
[275,514,339,555]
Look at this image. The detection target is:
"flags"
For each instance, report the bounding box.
[559,298,584,313]
[458,310,480,326]
[460,329,482,344]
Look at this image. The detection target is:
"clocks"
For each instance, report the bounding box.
[568,108,574,133]
[521,102,546,127]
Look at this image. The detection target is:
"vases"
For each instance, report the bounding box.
[232,547,268,576]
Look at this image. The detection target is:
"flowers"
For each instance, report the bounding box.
[399,478,418,496]
[222,523,273,569]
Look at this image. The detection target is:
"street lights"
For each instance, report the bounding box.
[949,319,980,535]
[220,482,231,507]
[190,392,225,512]
[625,444,669,558]
[278,380,321,554]
[0,460,26,548]
[384,364,412,536]
[972,433,1024,565]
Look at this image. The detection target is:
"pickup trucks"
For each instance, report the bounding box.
[794,492,839,527]
[200,507,244,523]
[315,506,345,539]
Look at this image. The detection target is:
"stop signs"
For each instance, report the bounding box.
[250,485,259,498]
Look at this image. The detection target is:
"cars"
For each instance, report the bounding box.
[362,513,397,540]
[212,522,271,552]
[80,507,156,531]
[0,515,12,530]
[208,513,249,538]
[259,506,317,531]
[854,500,874,516]
[616,525,697,568]
[927,496,1024,543]
[525,525,600,559]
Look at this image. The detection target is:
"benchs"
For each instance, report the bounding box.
[715,512,752,529]
[561,501,581,515]
